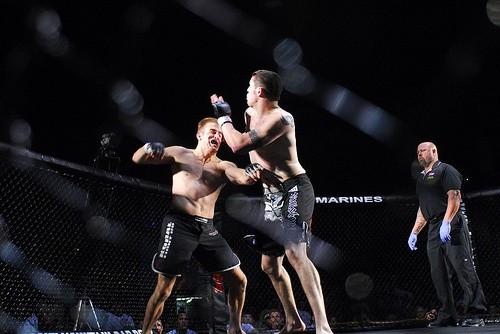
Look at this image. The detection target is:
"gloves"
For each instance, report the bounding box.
[440,221,451,242]
[407,233,418,251]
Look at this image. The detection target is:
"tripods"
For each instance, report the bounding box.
[73,149,121,332]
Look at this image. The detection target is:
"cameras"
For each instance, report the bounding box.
[100,132,122,148]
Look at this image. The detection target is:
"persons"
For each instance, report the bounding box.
[131,117,264,334]
[407,141,489,327]
[211,70,333,334]
[0,272,500,334]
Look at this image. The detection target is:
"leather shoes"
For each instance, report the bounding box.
[428,319,459,328]
[461,318,486,327]
